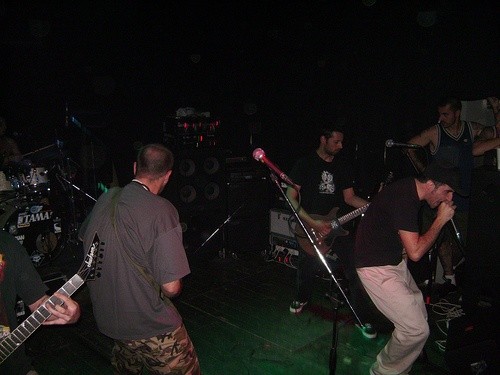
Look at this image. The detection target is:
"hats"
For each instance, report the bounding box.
[431,164,468,199]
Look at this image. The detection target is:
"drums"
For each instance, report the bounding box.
[1,198,65,268]
[20,163,58,198]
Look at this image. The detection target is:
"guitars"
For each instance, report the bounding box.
[295,202,370,256]
[0,242,105,366]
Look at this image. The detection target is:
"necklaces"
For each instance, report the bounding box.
[134,181,151,193]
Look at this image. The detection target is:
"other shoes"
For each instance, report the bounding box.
[354,320,378,338]
[290,300,309,313]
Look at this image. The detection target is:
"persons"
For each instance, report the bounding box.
[352,161,468,375]
[0,228,81,375]
[405,95,500,287]
[285,122,376,339]
[0,114,22,165]
[77,143,201,375]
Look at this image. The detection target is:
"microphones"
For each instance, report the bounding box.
[450,217,463,242]
[65,105,68,127]
[385,140,421,148]
[253,148,297,187]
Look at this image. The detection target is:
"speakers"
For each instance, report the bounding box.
[168,143,226,212]
